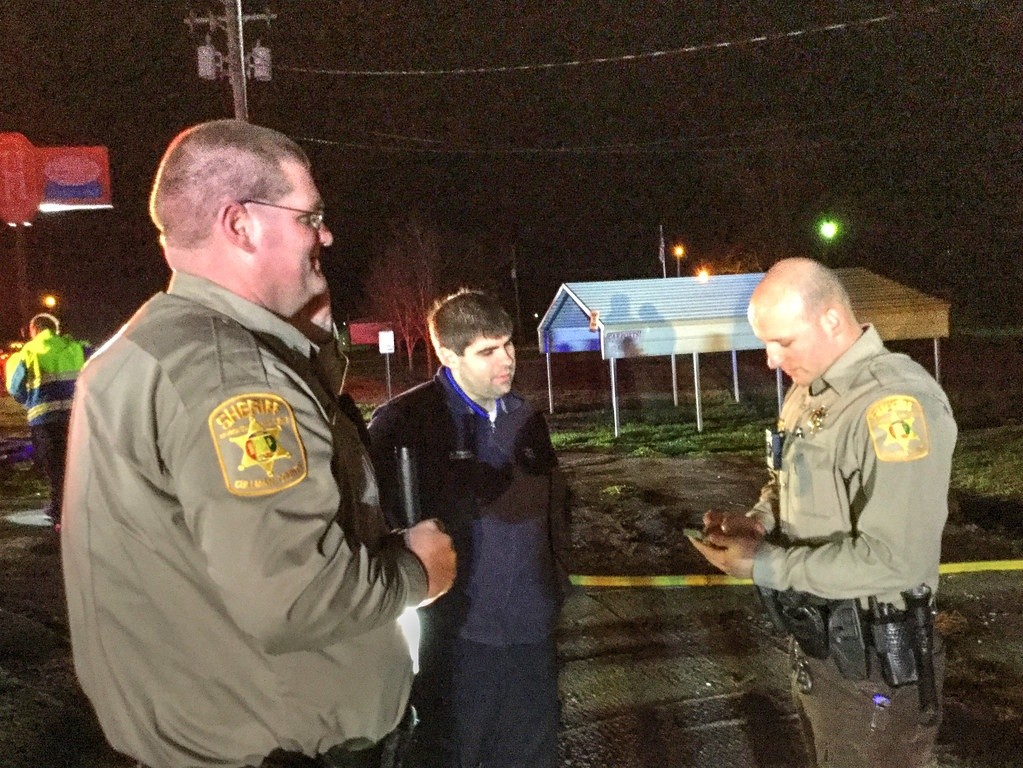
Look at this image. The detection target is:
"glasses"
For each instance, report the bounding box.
[237,199,325,230]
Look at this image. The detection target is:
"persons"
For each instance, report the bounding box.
[688,258,959,768]
[5,317,93,519]
[60,121,458,768]
[367,291,570,768]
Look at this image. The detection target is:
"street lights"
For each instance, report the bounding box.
[673,242,686,277]
[196,28,273,125]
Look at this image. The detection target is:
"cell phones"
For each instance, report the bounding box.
[681,527,707,539]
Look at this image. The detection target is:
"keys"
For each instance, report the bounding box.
[796,667,812,695]
